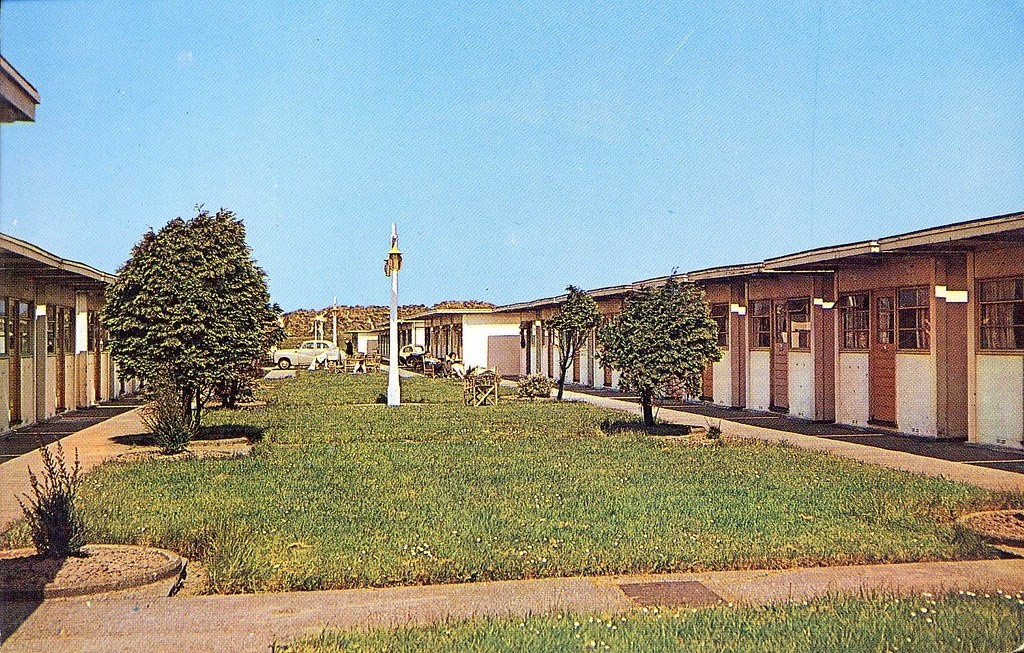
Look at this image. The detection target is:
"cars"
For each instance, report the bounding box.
[399,343,427,368]
[270,340,348,370]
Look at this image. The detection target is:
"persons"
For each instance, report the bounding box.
[447,352,466,380]
[344,338,353,356]
[424,351,449,377]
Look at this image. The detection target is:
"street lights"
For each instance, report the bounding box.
[331,299,339,348]
[384,221,403,406]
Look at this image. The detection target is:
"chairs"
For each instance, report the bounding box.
[330,351,381,374]
[422,354,463,378]
